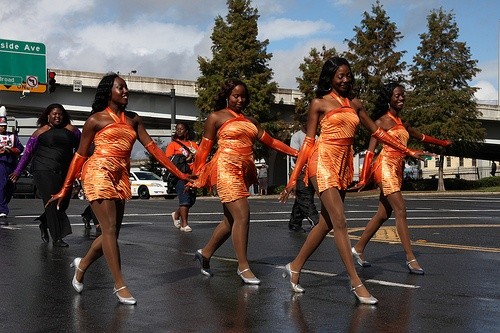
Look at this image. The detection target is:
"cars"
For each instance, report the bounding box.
[129,167,178,200]
[12,164,42,199]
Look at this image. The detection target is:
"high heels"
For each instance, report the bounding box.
[237,266,260,284]
[406,259,424,274]
[193,248,212,277]
[70,257,85,293]
[349,282,379,304]
[282,263,305,292]
[113,285,137,306]
[351,247,372,268]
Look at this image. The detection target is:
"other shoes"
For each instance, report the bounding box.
[82,217,92,229]
[95,225,102,232]
[0,213,7,217]
[180,225,193,232]
[39,224,49,242]
[171,212,180,228]
[289,225,307,232]
[52,240,69,247]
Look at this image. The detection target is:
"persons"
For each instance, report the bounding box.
[350,82,455,275]
[0,106,25,216]
[491,160,497,178]
[187,80,299,284]
[259,164,268,196]
[45,73,196,303]
[166,120,200,232]
[80,204,100,232]
[287,116,324,234]
[277,57,437,305]
[9,103,80,247]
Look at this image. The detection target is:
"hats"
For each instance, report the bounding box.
[0,106,9,126]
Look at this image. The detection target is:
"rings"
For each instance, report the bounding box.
[284,190,287,193]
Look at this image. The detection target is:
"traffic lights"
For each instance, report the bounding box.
[48,70,57,93]
